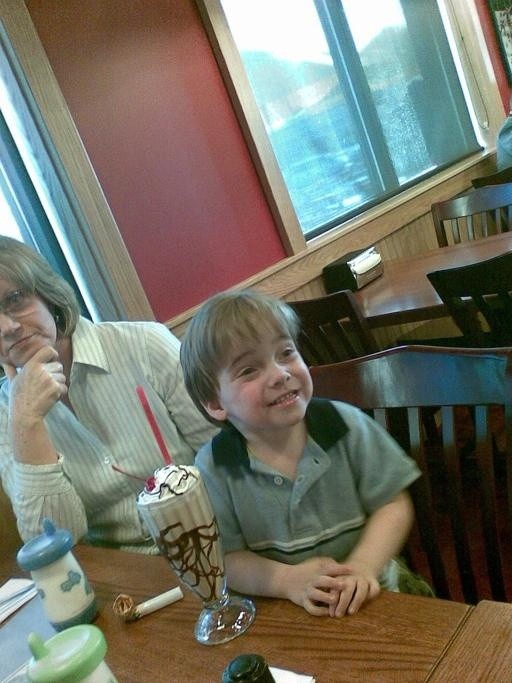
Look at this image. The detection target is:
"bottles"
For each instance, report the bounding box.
[16,519,99,632]
[25,622,119,683]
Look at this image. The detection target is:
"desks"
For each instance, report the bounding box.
[428,597,510,677]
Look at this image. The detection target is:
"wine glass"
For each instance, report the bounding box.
[136,473,257,648]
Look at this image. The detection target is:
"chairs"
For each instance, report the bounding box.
[309,344,512,604]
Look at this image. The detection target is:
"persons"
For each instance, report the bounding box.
[179,289,436,617]
[1,235,223,556]
[496,95,512,171]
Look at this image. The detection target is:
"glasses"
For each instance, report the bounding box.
[0,288,32,313]
